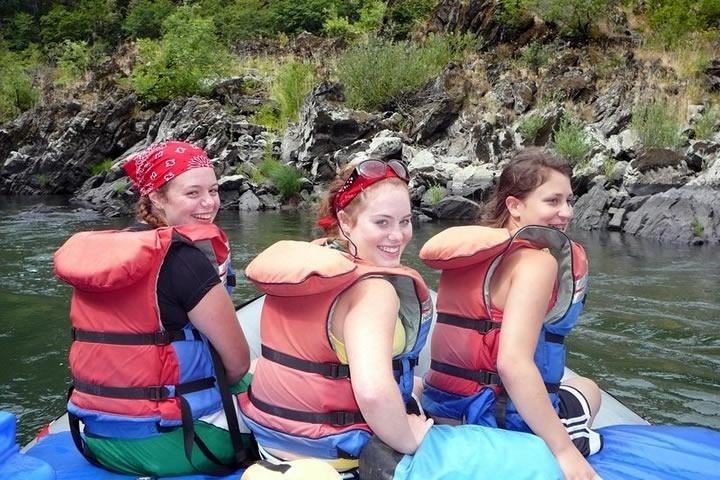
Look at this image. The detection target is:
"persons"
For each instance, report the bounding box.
[231,155,440,468]
[418,143,613,480]
[49,141,264,475]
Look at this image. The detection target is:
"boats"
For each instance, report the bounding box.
[18,288,652,480]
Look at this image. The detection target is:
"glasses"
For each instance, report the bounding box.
[332,158,411,207]
